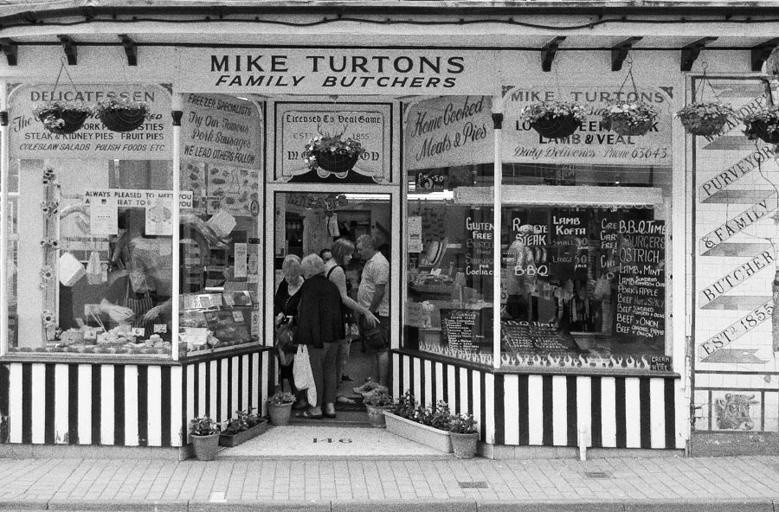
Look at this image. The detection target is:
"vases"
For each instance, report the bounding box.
[751,119,779,143]
[610,117,652,135]
[303,137,365,169]
[313,151,362,172]
[689,114,728,135]
[674,102,735,136]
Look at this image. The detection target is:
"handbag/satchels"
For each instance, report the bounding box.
[278,326,309,391]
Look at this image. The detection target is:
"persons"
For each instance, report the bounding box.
[273,221,391,421]
[101,260,172,343]
[505,223,539,322]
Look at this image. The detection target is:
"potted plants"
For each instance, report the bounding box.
[32,101,97,135]
[189,392,297,460]
[364,390,479,460]
[95,96,150,133]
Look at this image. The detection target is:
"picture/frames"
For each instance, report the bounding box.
[531,118,581,138]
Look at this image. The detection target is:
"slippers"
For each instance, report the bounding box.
[296,410,336,419]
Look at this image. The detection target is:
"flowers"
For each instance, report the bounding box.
[602,102,657,135]
[741,106,779,139]
[520,102,594,125]
